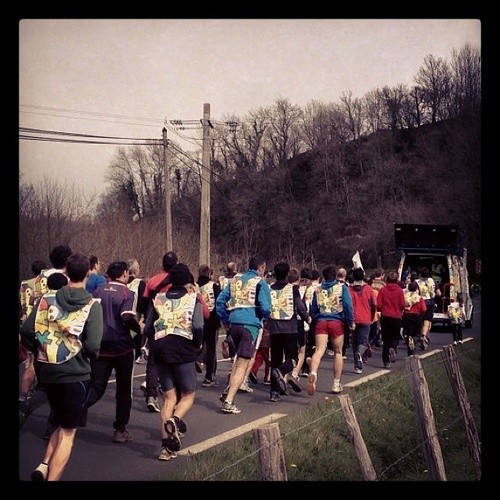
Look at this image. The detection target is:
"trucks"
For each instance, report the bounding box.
[393,221,477,332]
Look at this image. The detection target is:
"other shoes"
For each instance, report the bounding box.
[249,371,258,384]
[453,340,463,346]
[140,381,146,396]
[328,351,348,360]
[158,418,181,459]
[31,462,48,481]
[147,396,161,413]
[238,375,253,393]
[264,381,271,386]
[19,395,32,401]
[112,428,134,443]
[288,374,303,392]
[270,368,288,401]
[201,378,220,387]
[362,343,373,363]
[221,341,229,359]
[408,337,431,358]
[384,348,396,369]
[219,386,242,414]
[331,383,343,393]
[307,371,317,396]
[354,352,363,374]
[195,361,203,373]
[302,357,312,377]
[135,347,148,363]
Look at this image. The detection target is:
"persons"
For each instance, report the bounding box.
[145,263,205,460]
[216,255,272,413]
[269,263,311,402]
[196,263,222,387]
[336,267,443,374]
[83,261,145,443]
[20,243,74,439]
[307,265,355,395]
[84,252,210,437]
[446,299,463,346]
[21,252,103,482]
[219,261,352,392]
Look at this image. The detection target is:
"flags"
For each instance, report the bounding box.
[352,252,365,274]
